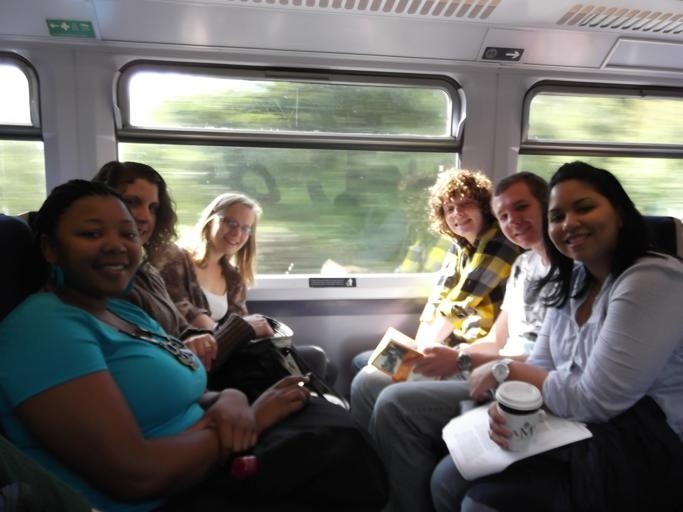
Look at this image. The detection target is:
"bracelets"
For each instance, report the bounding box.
[492,357,513,383]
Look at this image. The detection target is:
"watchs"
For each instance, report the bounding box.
[456,351,472,378]
[428,161,683,511]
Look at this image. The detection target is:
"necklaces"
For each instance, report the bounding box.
[117,323,198,370]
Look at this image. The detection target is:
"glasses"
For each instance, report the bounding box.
[214,215,255,235]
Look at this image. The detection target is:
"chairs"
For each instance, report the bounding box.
[0,212,109,510]
[639,215,683,260]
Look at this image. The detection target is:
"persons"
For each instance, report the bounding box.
[0,178,311,511]
[93,161,308,399]
[348,168,527,376]
[160,192,339,385]
[350,171,558,511]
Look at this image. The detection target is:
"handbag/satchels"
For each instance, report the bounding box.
[244,305,354,415]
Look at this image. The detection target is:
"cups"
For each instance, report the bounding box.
[494,379,544,453]
